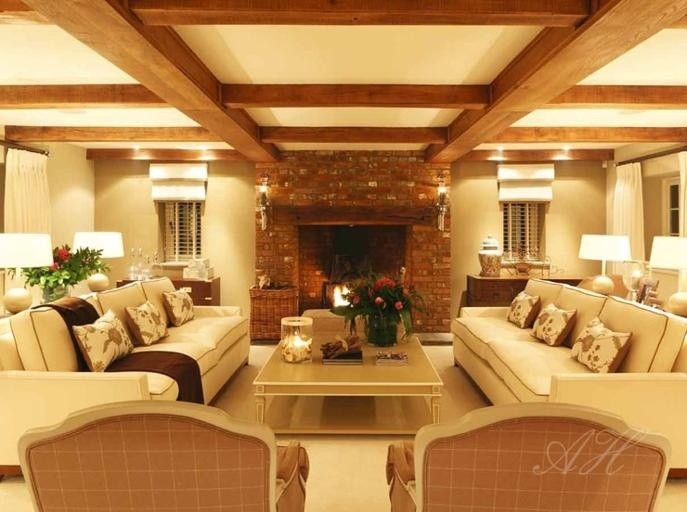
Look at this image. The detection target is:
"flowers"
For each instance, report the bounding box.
[24,247,105,285]
[330,267,433,340]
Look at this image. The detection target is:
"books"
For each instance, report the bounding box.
[322,350,408,367]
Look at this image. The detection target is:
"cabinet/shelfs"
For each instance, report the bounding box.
[115,275,223,305]
[466,272,598,307]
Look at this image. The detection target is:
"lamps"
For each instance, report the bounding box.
[0,229,54,315]
[436,168,447,206]
[578,230,628,293]
[73,229,125,292]
[257,172,272,207]
[649,234,687,316]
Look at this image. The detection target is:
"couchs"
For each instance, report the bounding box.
[455,276,687,476]
[2,276,252,484]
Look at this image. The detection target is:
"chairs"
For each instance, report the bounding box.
[383,402,674,512]
[16,399,309,512]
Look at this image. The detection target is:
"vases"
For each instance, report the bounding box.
[365,316,399,346]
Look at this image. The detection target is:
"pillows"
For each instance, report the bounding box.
[165,289,196,324]
[507,294,537,328]
[532,303,578,343]
[573,318,632,375]
[69,308,131,372]
[122,298,168,347]
